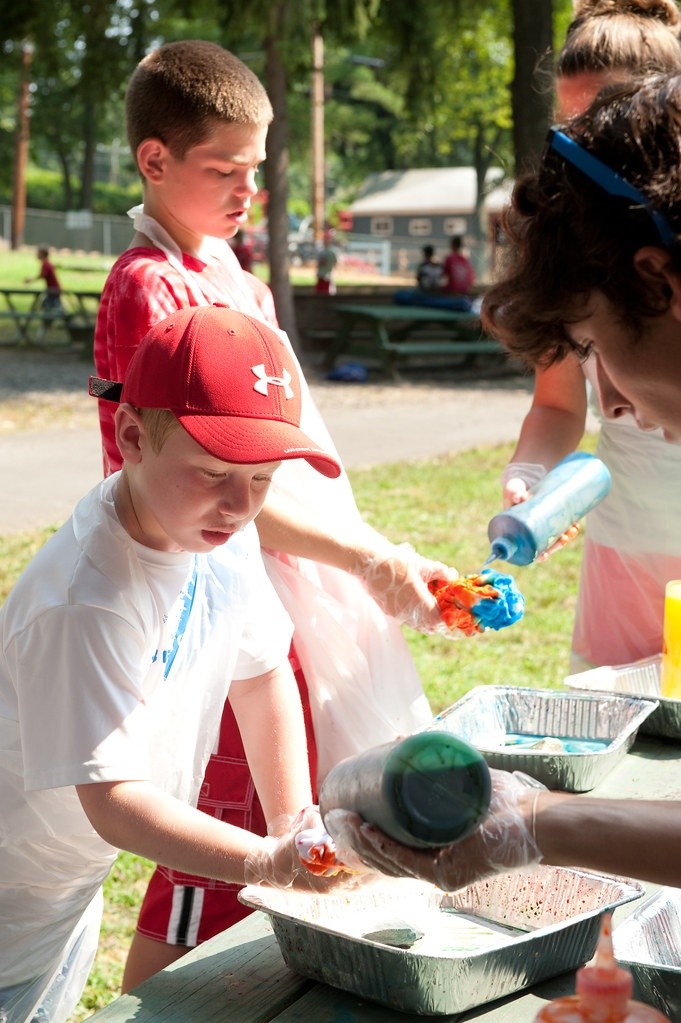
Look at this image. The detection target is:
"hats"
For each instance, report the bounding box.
[88,302,342,479]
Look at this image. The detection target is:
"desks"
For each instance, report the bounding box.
[68,704,681,1023]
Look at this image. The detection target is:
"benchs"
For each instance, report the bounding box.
[303,303,513,385]
[0,287,105,351]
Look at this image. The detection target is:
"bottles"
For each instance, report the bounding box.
[660,580,679,700]
[317,726,493,851]
[484,448,611,566]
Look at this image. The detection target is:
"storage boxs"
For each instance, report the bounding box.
[602,882,681,1023]
[416,681,661,794]
[562,651,681,746]
[236,847,646,1018]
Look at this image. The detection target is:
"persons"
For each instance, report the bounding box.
[418,244,446,294]
[24,245,78,345]
[497,0,679,678]
[92,39,319,989]
[442,232,472,293]
[231,229,255,273]
[319,235,338,296]
[326,76,681,893]
[2,303,347,1023]
